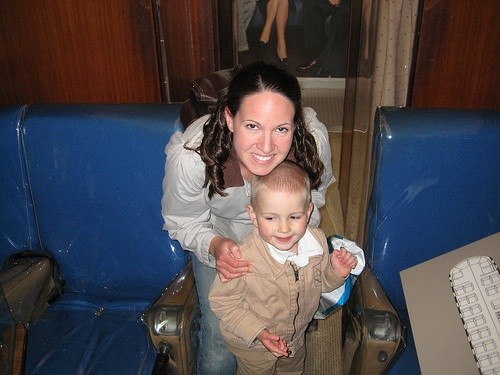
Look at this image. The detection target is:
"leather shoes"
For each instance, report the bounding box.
[296,63,326,72]
[313,69,336,77]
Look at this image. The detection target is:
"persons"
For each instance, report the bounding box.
[257,0,296,66]
[162,60,337,375]
[210,159,358,375]
[236,0,353,73]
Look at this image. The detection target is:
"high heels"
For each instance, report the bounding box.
[258,34,271,48]
[272,42,288,67]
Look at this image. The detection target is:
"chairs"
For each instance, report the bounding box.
[0,102,500,374]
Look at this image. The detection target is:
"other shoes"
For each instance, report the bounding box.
[247,49,262,61]
[235,52,246,64]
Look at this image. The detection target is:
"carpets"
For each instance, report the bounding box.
[301,89,346,133]
